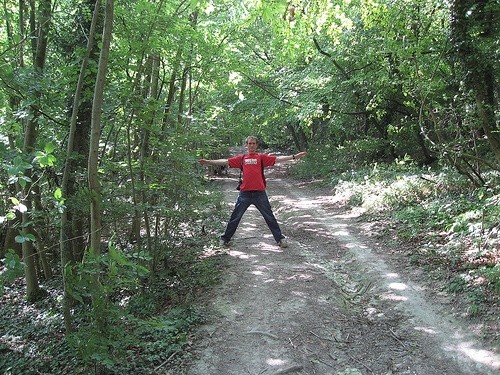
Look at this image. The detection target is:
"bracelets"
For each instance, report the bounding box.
[293,154,295,159]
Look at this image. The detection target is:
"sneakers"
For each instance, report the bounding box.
[219,239,226,248]
[276,238,288,248]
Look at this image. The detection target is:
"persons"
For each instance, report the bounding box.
[198,135,308,248]
